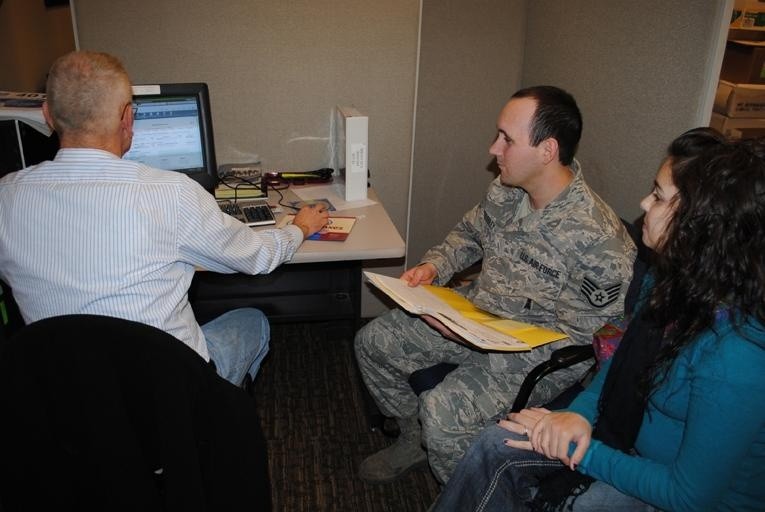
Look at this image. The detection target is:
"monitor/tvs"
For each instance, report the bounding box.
[121,82,218,189]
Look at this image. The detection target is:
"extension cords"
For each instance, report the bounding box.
[218,163,262,179]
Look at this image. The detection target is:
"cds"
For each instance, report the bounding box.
[294,201,330,211]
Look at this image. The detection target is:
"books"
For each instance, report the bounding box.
[214,181,263,198]
[362,267,569,350]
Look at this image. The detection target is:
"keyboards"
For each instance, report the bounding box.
[216,200,276,227]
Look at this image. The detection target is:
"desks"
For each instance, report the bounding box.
[188,170,407,326]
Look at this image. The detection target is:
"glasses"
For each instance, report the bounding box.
[120,102,140,120]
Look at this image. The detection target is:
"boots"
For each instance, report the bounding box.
[358,415,428,484]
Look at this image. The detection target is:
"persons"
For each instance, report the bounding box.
[353,86,638,492]
[0,50,329,391]
[429,126,765,512]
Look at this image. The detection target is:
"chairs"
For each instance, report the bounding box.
[409,217,650,418]
[2,315,251,511]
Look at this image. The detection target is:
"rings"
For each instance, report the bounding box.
[523,424,528,436]
